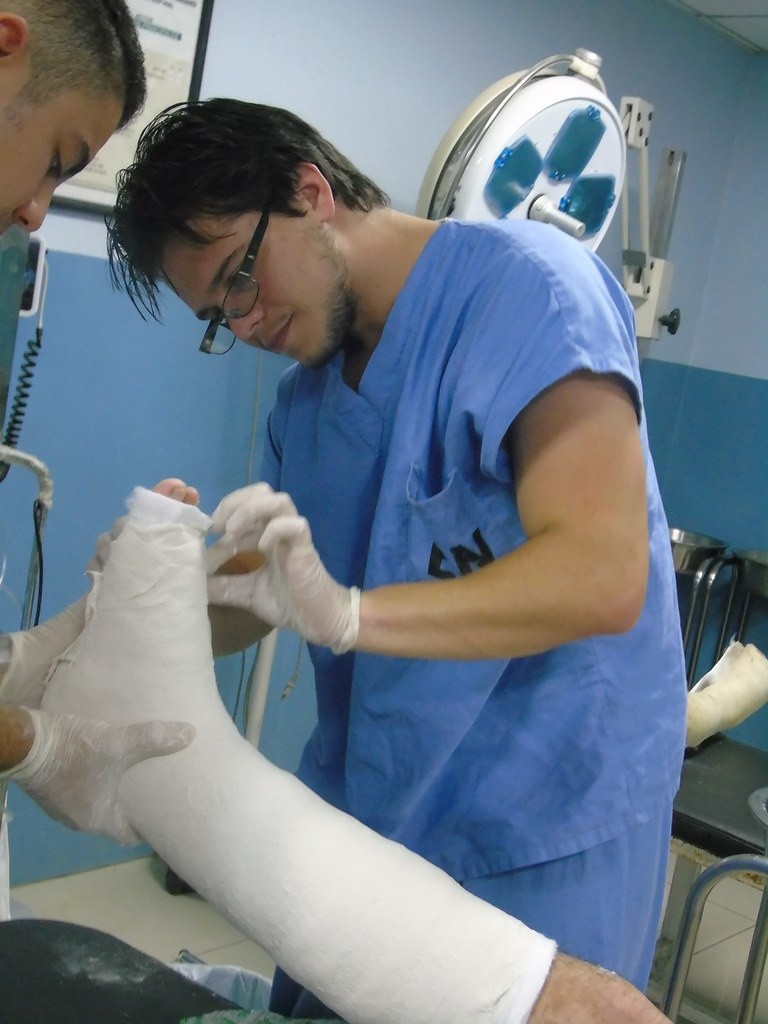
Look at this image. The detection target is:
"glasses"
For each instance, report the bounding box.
[199,186,277,354]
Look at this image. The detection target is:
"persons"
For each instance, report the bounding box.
[0,0,195,851]
[36,478,675,1021]
[101,96,688,1024]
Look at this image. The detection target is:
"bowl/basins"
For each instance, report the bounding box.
[737,548,768,597]
[669,529,727,577]
